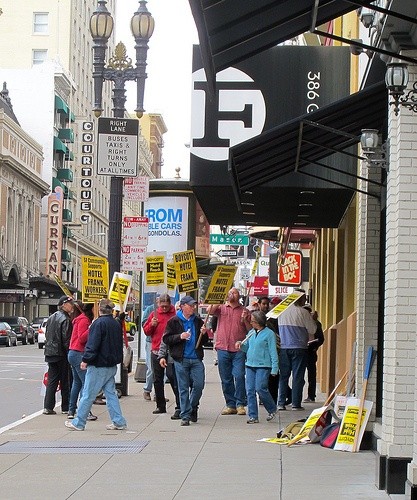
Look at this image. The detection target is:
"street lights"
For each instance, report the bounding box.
[88,1,155,282]
[73,232,106,301]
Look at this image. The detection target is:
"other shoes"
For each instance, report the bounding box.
[170,409,182,420]
[85,412,97,421]
[60,408,69,415]
[302,397,315,403]
[221,407,237,416]
[93,398,106,406]
[67,412,75,419]
[152,396,169,402]
[43,408,56,415]
[190,407,200,423]
[152,406,167,414]
[236,405,247,415]
[180,418,190,426]
[291,406,305,411]
[143,391,151,400]
[276,406,286,411]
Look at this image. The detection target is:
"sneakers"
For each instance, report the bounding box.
[266,408,279,422]
[246,417,259,424]
[64,421,84,431]
[105,422,127,430]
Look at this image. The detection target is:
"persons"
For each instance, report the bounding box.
[212,290,325,410]
[235,311,279,423]
[126,316,131,322]
[163,296,208,425]
[64,298,126,430]
[140,294,194,419]
[44,296,106,420]
[207,288,250,414]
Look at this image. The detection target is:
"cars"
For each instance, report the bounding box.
[124,319,137,336]
[0,315,50,349]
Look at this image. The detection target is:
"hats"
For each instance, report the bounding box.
[57,295,80,306]
[179,295,197,306]
[251,302,257,306]
[270,297,282,305]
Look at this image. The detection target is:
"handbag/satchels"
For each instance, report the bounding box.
[40,371,61,392]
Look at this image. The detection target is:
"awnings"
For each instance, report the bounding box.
[188,0,375,98]
[226,80,388,212]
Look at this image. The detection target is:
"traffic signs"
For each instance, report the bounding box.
[216,249,238,257]
